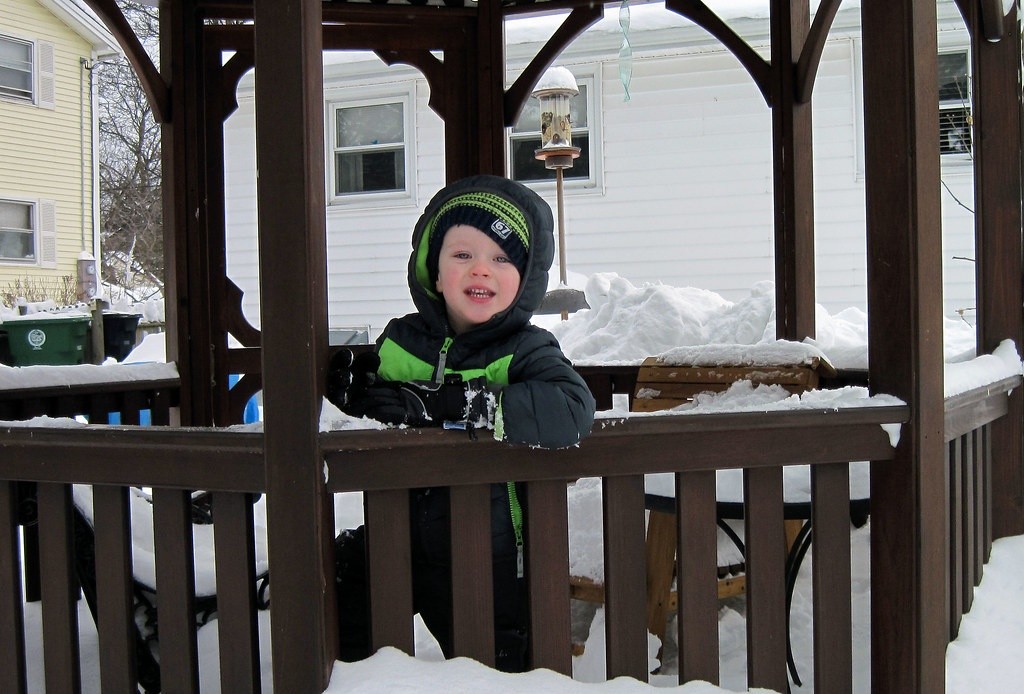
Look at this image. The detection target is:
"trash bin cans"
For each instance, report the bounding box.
[0,317,94,369]
[101,314,143,362]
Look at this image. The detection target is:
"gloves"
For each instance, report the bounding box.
[324,348,380,417]
[364,382,445,424]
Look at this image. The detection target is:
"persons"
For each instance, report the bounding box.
[325,174,597,673]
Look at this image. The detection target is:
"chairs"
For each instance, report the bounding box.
[570,341,868,686]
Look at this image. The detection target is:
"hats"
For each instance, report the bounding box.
[427,191,531,281]
